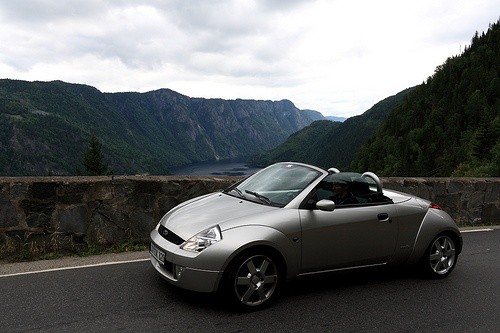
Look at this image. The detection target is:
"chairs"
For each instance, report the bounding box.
[313,172,369,206]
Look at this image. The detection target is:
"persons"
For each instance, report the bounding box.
[317,173,359,204]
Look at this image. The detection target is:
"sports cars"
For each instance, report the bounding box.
[148,161,464,313]
